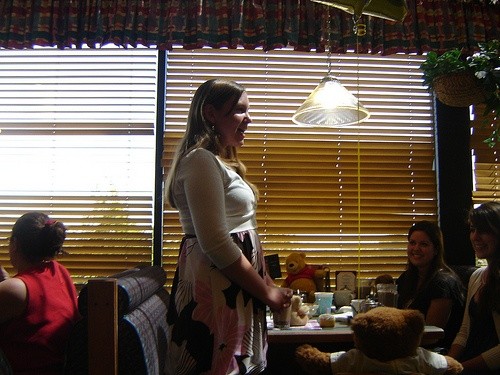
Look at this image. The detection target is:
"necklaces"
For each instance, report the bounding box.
[482,268,499,285]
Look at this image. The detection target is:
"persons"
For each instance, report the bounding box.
[395,220,466,355]
[0,212,80,375]
[446,200,500,375]
[162,78,293,374]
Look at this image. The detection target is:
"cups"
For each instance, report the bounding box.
[314,292,334,315]
[378,292,398,307]
[335,291,353,309]
[273,303,291,328]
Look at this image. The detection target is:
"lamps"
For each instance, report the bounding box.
[291,0,371,128]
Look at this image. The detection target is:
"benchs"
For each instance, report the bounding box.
[65,261,174,375]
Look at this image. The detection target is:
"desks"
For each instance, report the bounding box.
[266,311,445,375]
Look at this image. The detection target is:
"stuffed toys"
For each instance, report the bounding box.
[296,306,463,375]
[281,252,327,303]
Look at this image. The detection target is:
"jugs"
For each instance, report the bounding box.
[349,296,383,314]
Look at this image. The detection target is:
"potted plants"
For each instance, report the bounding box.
[420,39,500,148]
